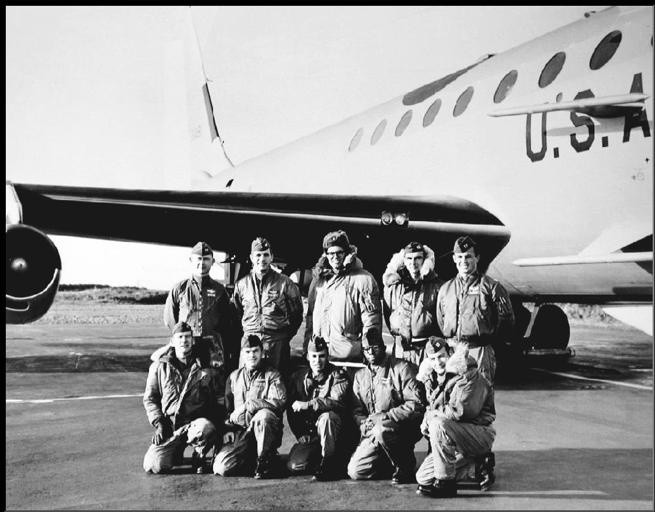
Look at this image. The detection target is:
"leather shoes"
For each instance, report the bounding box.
[416,481,457,498]
[476,453,495,491]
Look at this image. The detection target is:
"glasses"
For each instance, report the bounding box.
[325,251,344,256]
[362,347,380,353]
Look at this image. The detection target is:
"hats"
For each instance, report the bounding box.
[405,241,423,253]
[307,335,327,351]
[453,236,473,252]
[172,321,191,332]
[362,327,382,346]
[322,230,349,249]
[425,335,444,354]
[240,334,261,348]
[191,241,213,256]
[251,236,270,251]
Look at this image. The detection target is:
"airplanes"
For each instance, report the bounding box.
[0,7,654,379]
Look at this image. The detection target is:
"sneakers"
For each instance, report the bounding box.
[191,452,206,474]
[254,456,271,478]
[392,463,406,482]
[313,457,334,481]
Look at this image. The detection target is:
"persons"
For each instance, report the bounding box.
[436,237,513,392]
[416,337,495,497]
[377,240,442,453]
[142,321,216,475]
[164,241,229,376]
[346,326,428,483]
[301,228,376,388]
[286,337,350,480]
[211,334,287,478]
[229,235,304,377]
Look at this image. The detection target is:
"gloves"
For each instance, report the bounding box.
[152,421,165,446]
